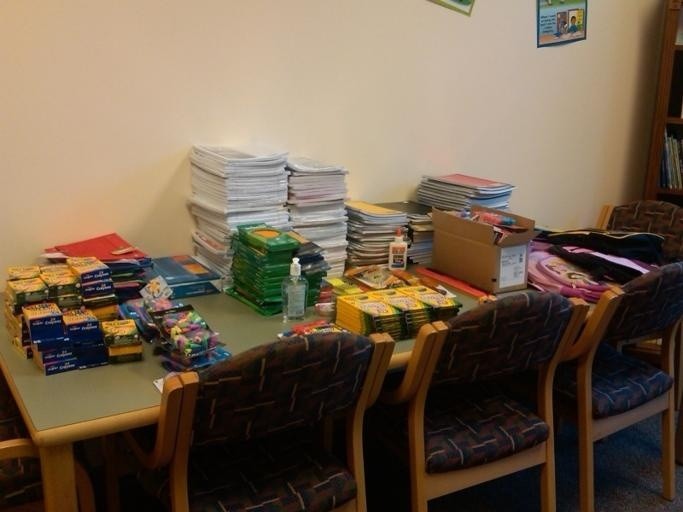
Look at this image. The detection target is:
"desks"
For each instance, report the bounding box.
[0,266,599,512]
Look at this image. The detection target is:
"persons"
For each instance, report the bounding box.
[568,16,577,32]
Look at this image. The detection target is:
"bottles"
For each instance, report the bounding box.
[387,229,409,271]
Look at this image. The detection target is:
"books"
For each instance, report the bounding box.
[657,128,683,191]
[325,268,460,341]
[45,233,224,300]
[184,146,351,317]
[344,175,517,269]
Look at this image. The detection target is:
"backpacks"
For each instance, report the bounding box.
[526,241,660,303]
